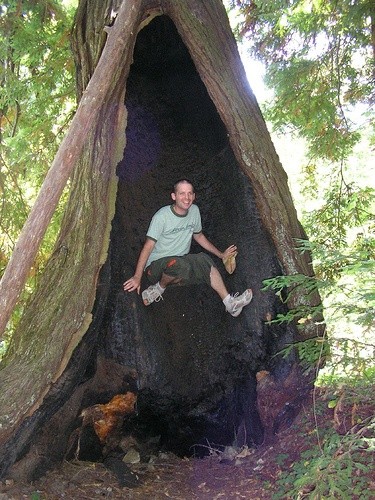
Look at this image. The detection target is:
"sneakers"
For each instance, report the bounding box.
[142,284,163,306]
[225,289,253,317]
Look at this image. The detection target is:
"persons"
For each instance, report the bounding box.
[123,179,253,317]
